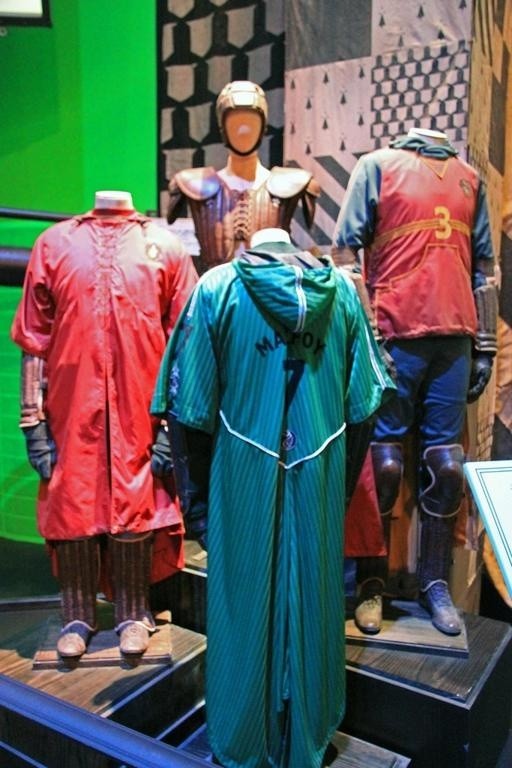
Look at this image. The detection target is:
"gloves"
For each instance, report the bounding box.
[150,431,174,478]
[469,359,492,401]
[25,424,59,479]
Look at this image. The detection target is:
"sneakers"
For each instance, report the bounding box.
[57,625,90,658]
[421,585,462,636]
[354,589,381,634]
[120,624,150,653]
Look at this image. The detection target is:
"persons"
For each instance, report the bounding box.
[166,76,325,277]
[145,224,401,767]
[331,126,502,638]
[10,188,203,663]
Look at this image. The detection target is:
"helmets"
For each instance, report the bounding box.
[218,82,267,156]
